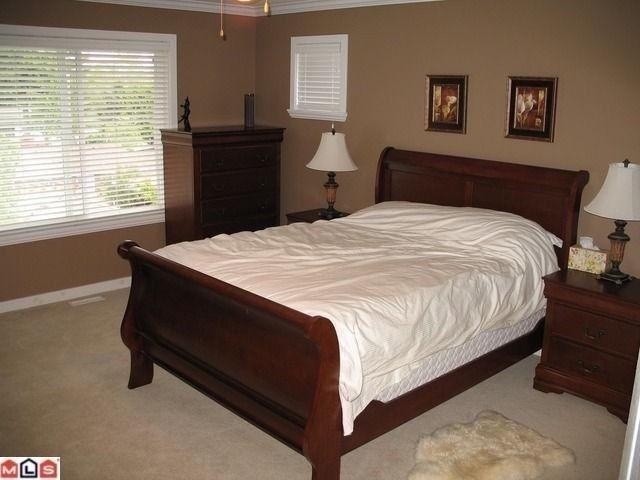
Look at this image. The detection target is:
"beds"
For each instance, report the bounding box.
[118,149,589,478]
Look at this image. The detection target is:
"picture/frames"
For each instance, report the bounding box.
[425,74,468,135]
[504,75,558,143]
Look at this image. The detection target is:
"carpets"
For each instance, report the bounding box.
[409,410,578,480]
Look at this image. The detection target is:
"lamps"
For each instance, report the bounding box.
[306,129,358,218]
[583,159,640,285]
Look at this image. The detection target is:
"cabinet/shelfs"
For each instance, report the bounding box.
[160,128,286,245]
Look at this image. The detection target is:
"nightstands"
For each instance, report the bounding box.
[285,209,349,223]
[533,268,639,420]
